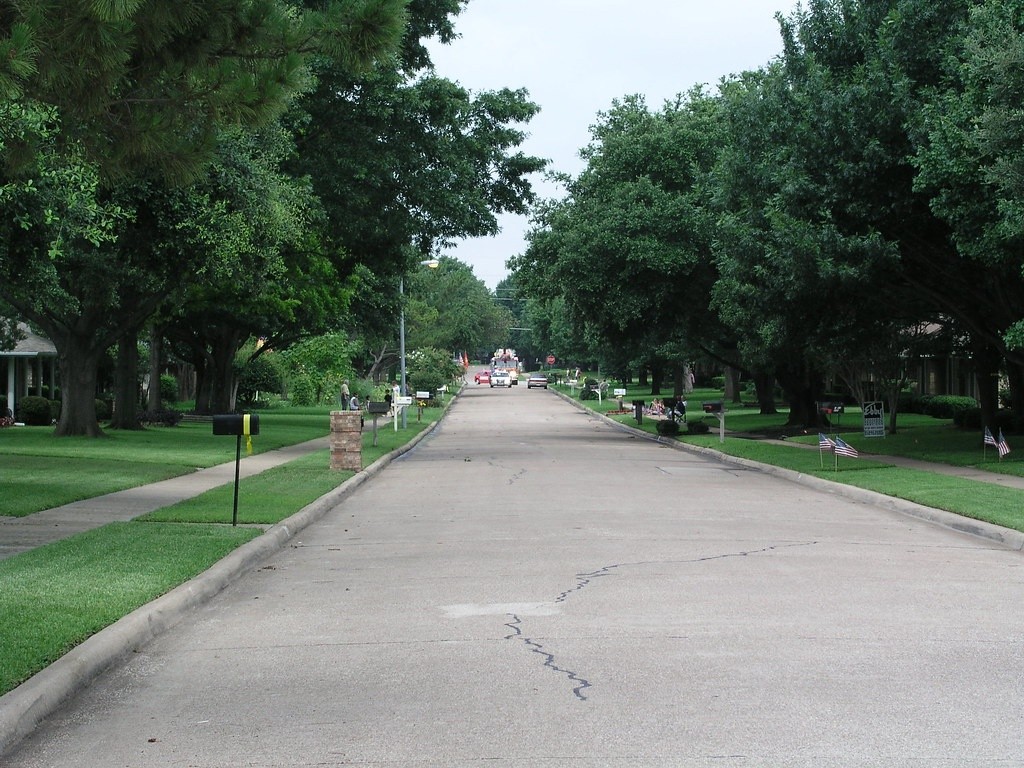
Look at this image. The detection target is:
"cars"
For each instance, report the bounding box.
[475,371,492,384]
[823,393,844,414]
[528,374,548,389]
[489,372,512,388]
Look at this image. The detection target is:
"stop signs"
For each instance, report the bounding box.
[548,356,555,364]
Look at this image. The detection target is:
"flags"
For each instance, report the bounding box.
[985,429,998,448]
[999,432,1010,455]
[460,352,469,371]
[835,436,858,458]
[819,433,832,449]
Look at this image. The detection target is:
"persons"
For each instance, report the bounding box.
[564,367,571,383]
[384,389,394,417]
[668,394,687,420]
[392,380,400,395]
[365,395,371,410]
[600,380,608,390]
[644,398,665,415]
[685,371,695,394]
[349,393,361,410]
[340,379,350,410]
[575,366,581,380]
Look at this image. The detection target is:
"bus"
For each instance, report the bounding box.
[490,349,518,384]
